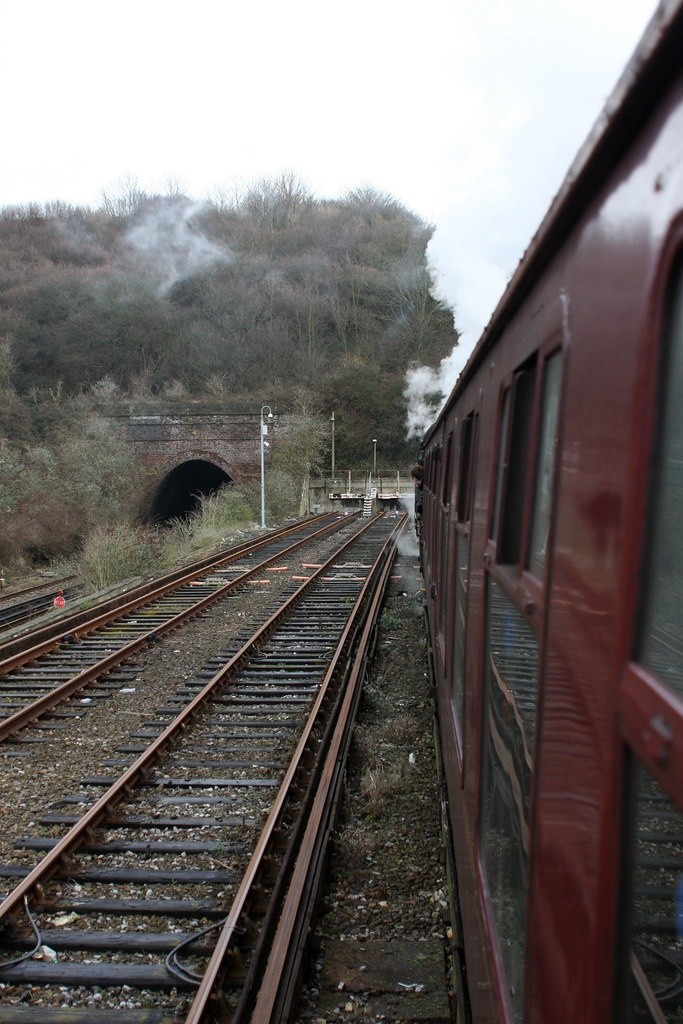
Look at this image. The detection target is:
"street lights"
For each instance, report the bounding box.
[372,438,377,477]
[259,406,273,528]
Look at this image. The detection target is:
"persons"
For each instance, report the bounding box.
[411,461,425,487]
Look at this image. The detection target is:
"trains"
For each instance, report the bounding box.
[409,0,682,1024]
